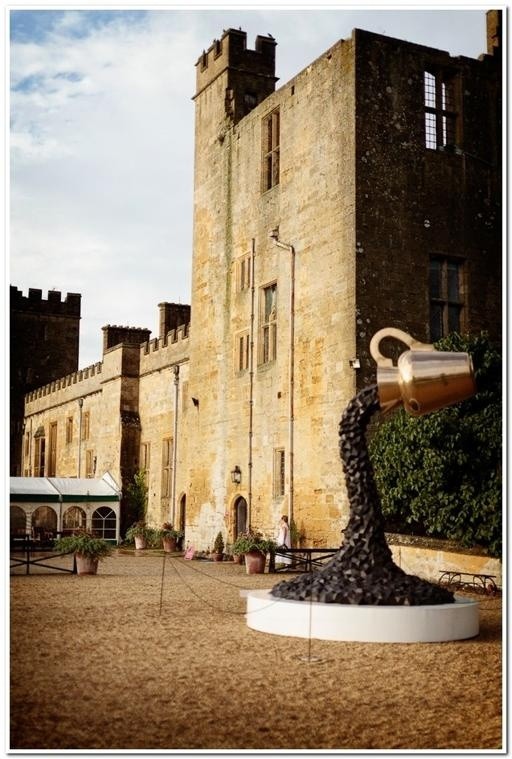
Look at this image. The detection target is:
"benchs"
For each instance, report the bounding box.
[436,570,500,597]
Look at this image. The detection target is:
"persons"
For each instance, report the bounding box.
[274,514,293,568]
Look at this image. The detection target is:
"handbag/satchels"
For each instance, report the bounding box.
[274,544,292,565]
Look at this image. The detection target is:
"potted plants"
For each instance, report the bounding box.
[210,530,226,562]
[155,527,186,554]
[231,532,272,574]
[127,526,154,549]
[230,532,244,567]
[54,536,114,577]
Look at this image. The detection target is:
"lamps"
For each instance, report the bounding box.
[231,463,241,485]
[190,397,198,407]
[78,397,84,408]
[173,363,179,373]
